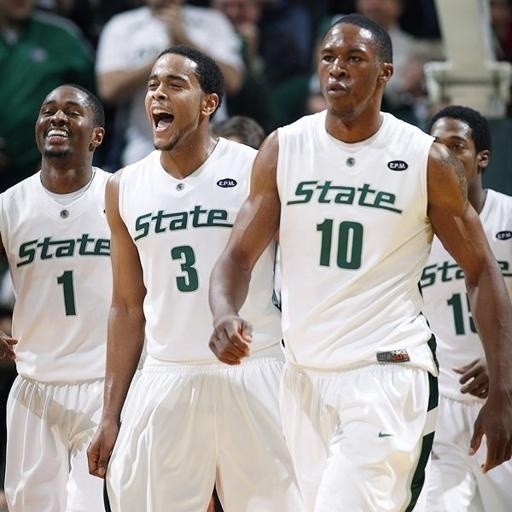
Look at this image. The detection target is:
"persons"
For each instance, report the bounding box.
[94,0,244,175]
[85,43,291,511]
[407,103,511,512]
[0,2,92,190]
[208,0,443,146]
[0,82,111,512]
[206,12,511,512]
[489,1,510,63]
